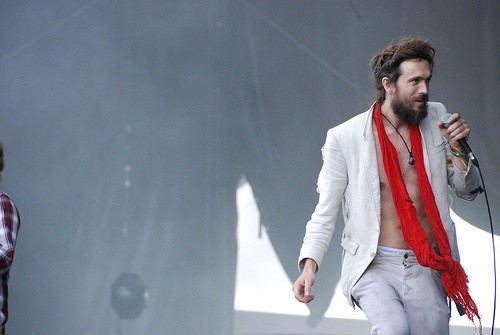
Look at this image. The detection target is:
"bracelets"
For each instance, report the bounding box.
[449,144,469,156]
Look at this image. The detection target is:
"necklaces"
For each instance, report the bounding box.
[380,110,419,165]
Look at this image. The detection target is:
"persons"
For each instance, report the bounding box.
[291,35,484,335]
[0,145,20,335]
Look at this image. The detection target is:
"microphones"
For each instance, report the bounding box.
[442,113,479,168]
